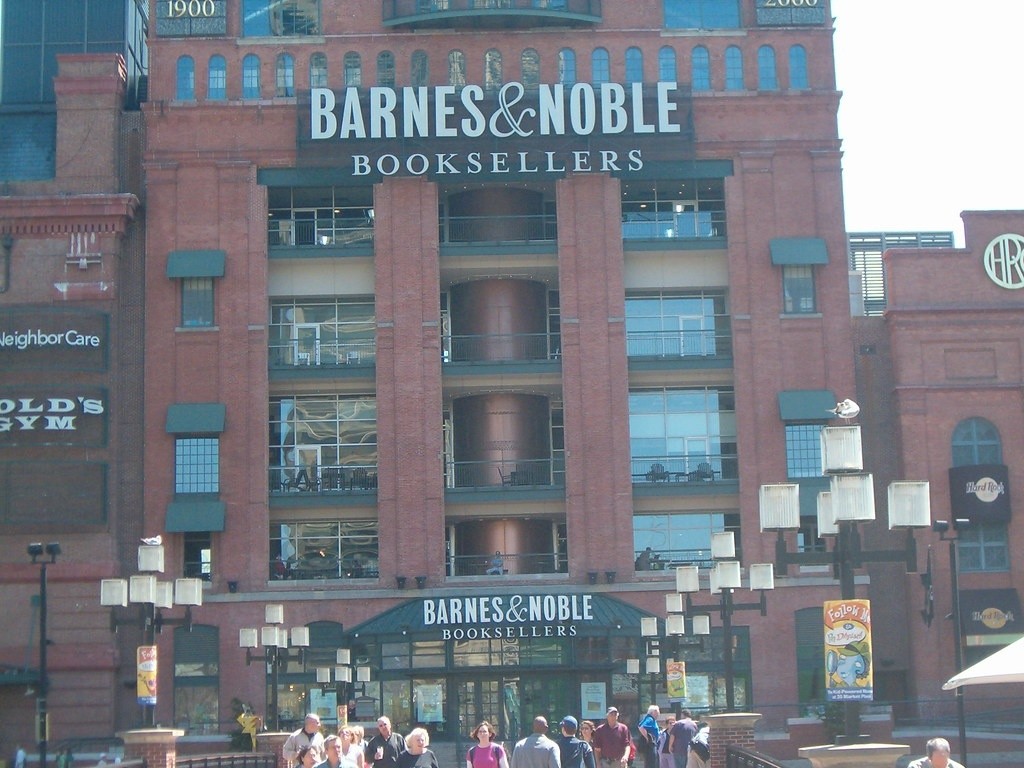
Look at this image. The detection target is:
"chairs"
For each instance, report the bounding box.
[650,463,669,483]
[297,353,310,365]
[347,351,361,364]
[281,469,377,491]
[498,467,512,486]
[697,463,714,481]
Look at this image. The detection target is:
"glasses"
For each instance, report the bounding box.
[580,726,591,731]
[379,722,389,727]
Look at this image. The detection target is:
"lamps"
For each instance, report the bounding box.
[227,581,237,593]
[396,574,425,590]
[589,570,615,584]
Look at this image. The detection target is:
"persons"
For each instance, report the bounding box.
[283,713,439,768]
[635,547,651,570]
[465,706,636,768]
[348,559,363,578]
[273,552,285,579]
[485,551,504,575]
[907,737,966,768]
[638,705,710,768]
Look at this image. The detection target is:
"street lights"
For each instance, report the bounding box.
[239,603,309,733]
[757,425,931,747]
[98,535,203,730]
[626,592,711,720]
[675,532,774,714]
[316,648,371,731]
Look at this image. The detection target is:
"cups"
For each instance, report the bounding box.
[377,746,384,760]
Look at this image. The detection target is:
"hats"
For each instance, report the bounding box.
[607,706,618,715]
[559,715,577,729]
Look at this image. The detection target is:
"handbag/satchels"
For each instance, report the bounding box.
[689,741,710,762]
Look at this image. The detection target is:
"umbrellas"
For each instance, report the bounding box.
[942,636,1024,691]
